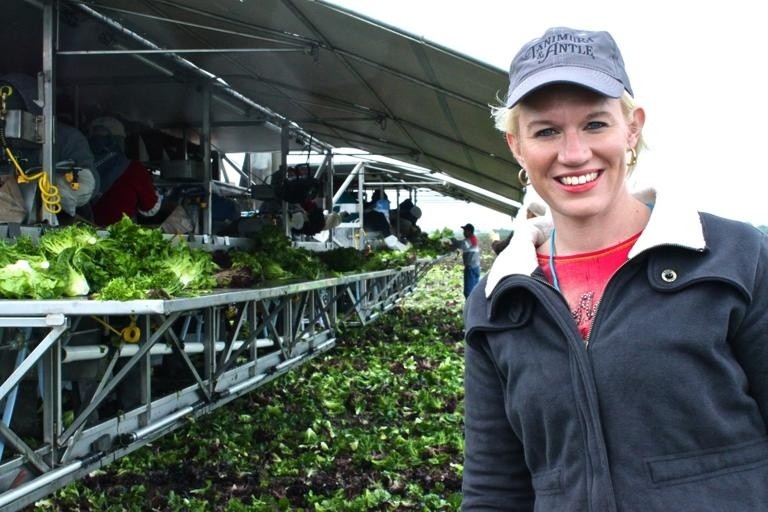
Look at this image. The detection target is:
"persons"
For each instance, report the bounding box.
[449,224,481,299]
[2,72,163,230]
[461,26,768,511]
[492,203,546,255]
[259,164,421,240]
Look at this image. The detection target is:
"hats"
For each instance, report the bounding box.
[506,26,634,110]
[88,116,129,139]
[461,224,474,232]
